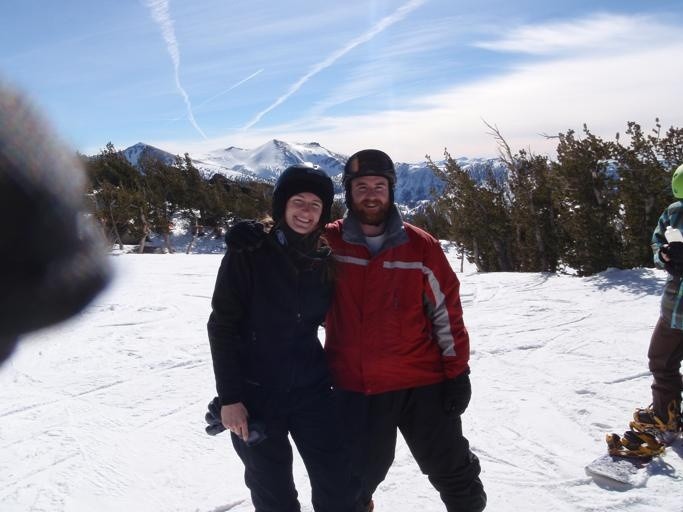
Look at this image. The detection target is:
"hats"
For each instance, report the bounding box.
[342,150,397,183]
[272,161,335,220]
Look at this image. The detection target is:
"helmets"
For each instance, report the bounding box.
[672,164,683,198]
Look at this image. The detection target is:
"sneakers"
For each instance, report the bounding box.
[637,409,681,425]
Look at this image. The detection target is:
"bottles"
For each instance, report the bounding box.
[664,225,682,246]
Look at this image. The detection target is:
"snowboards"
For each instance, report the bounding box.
[586,407,683,486]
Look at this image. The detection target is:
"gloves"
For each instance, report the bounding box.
[445,371,471,416]
[205,397,267,447]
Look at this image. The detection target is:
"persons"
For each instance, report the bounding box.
[199,161,339,510]
[316,150,489,511]
[0,85,118,366]
[630,163,683,446]
